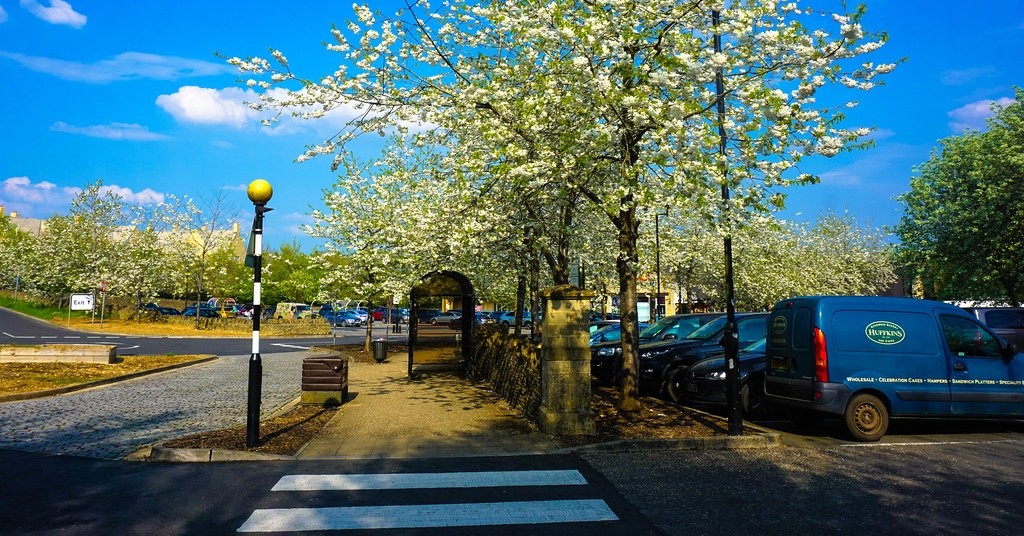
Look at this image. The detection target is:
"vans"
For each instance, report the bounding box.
[760,295,1024,441]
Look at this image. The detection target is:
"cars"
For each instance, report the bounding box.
[372,306,385,321]
[322,310,361,327]
[639,312,771,385]
[263,304,276,321]
[430,311,462,325]
[350,310,368,324]
[474,309,542,329]
[588,314,726,381]
[589,321,650,347]
[145,302,254,324]
[382,308,411,324]
[686,338,766,411]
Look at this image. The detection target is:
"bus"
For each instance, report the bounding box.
[275,301,310,324]
[207,297,236,308]
[418,308,441,322]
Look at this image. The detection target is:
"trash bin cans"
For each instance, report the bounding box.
[301,353,349,407]
[372,339,387,362]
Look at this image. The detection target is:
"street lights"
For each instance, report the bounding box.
[246,176,274,450]
